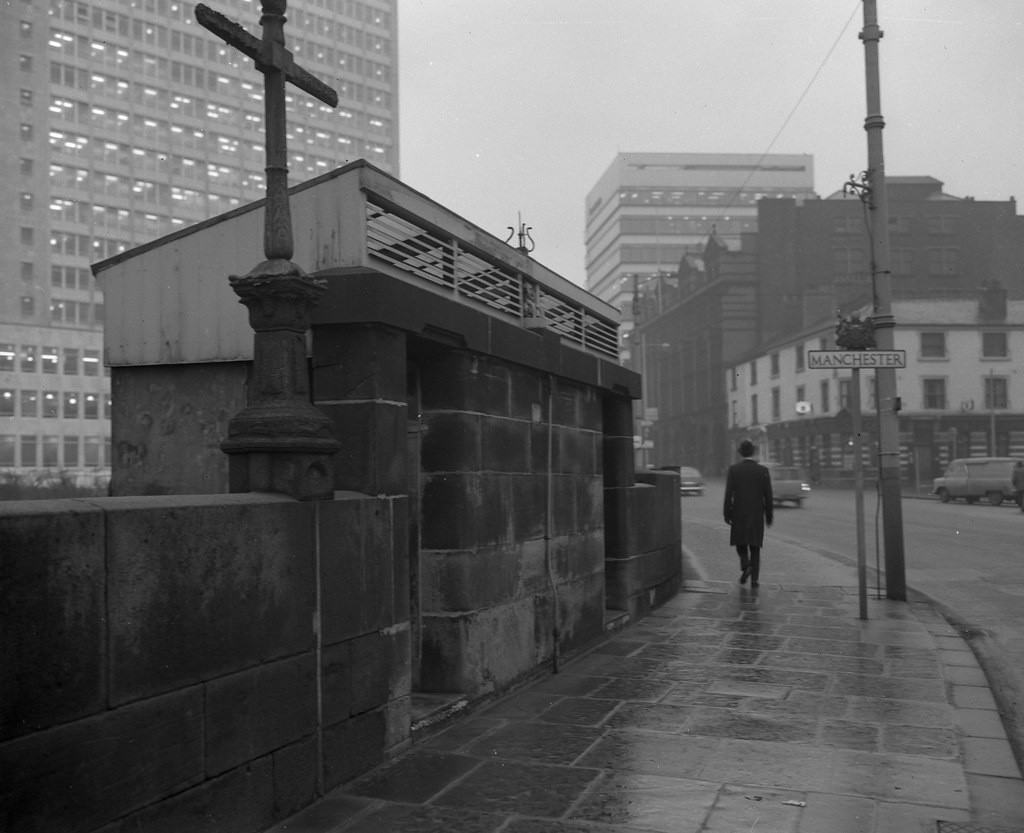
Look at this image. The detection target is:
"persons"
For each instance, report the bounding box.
[1011,462,1024,512]
[723,439,773,586]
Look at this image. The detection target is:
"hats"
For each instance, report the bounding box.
[739,441,754,454]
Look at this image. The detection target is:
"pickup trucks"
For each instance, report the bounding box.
[768,466,812,509]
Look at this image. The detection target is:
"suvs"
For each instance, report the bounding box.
[931,456,1022,506]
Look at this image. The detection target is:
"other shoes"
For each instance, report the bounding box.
[740,566,752,584]
[752,581,760,588]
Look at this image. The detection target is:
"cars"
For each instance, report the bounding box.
[679,466,708,495]
